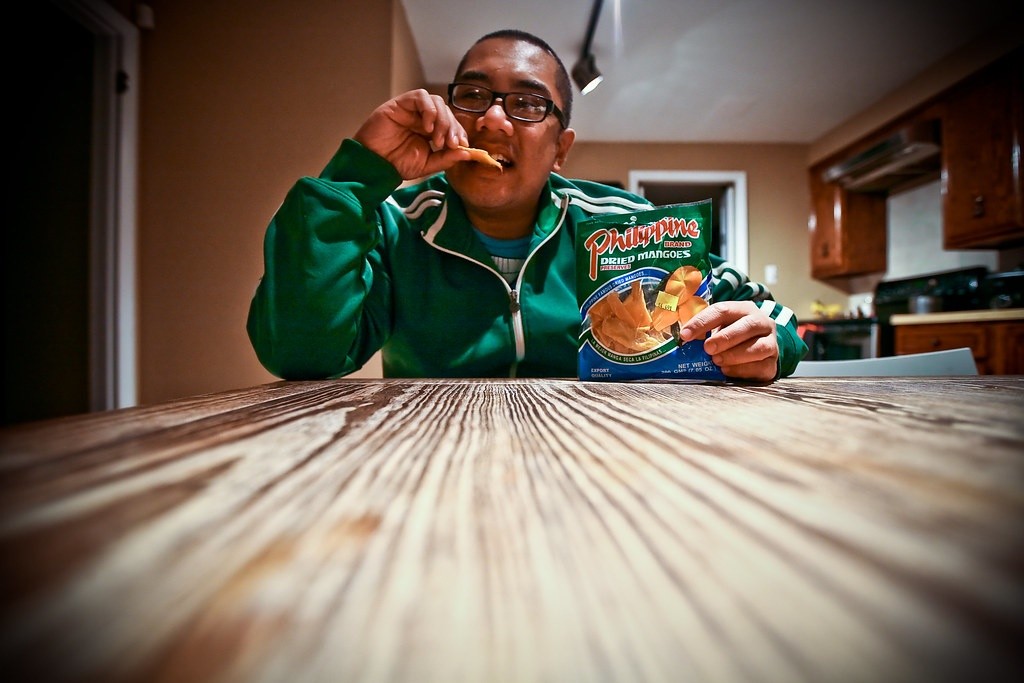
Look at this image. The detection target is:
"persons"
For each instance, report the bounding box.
[245,27,810,387]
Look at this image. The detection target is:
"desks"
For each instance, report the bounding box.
[0,377,1024,683]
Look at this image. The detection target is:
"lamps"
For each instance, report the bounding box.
[571,0,605,95]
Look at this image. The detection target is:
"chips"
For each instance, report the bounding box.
[458,146,503,174]
[591,266,710,353]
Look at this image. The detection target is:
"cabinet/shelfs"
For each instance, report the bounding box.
[895,318,1023,374]
[808,142,886,281]
[940,44,1023,250]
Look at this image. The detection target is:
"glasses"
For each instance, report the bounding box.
[447,83,567,130]
